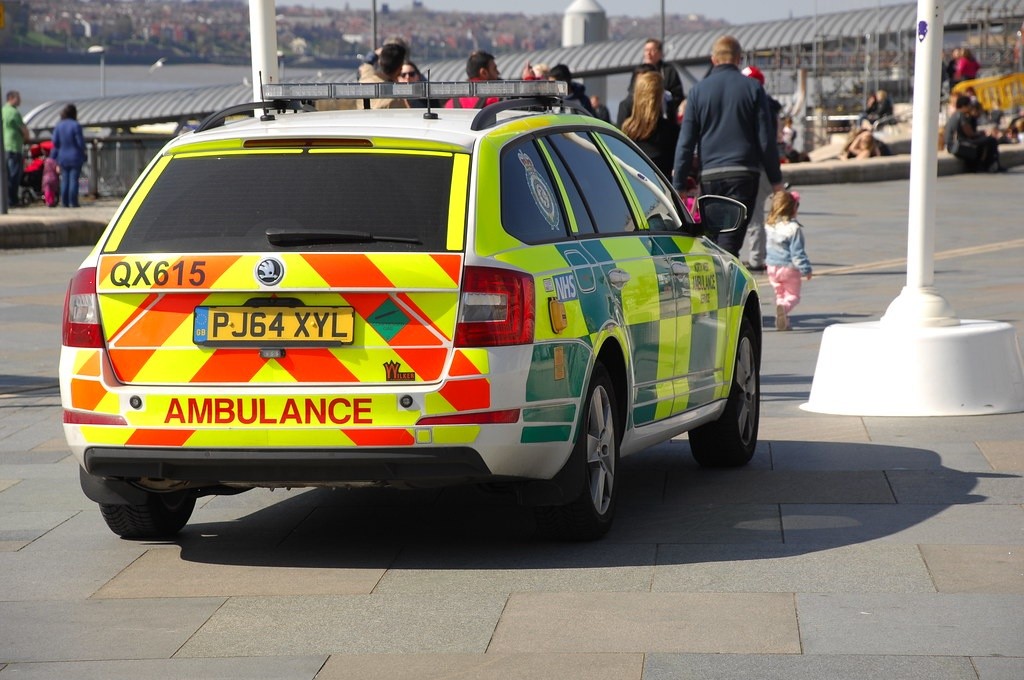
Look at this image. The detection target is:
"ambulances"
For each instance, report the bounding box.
[58,68,764,547]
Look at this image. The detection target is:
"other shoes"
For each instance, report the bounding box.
[62,204,80,207]
[48,204,57,207]
[775,304,791,330]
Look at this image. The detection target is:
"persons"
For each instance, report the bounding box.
[1,90,87,209]
[837,47,1024,172]
[356,36,813,331]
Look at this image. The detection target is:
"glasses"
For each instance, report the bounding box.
[400,71,417,78]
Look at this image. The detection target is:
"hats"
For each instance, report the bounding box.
[741,66,764,85]
